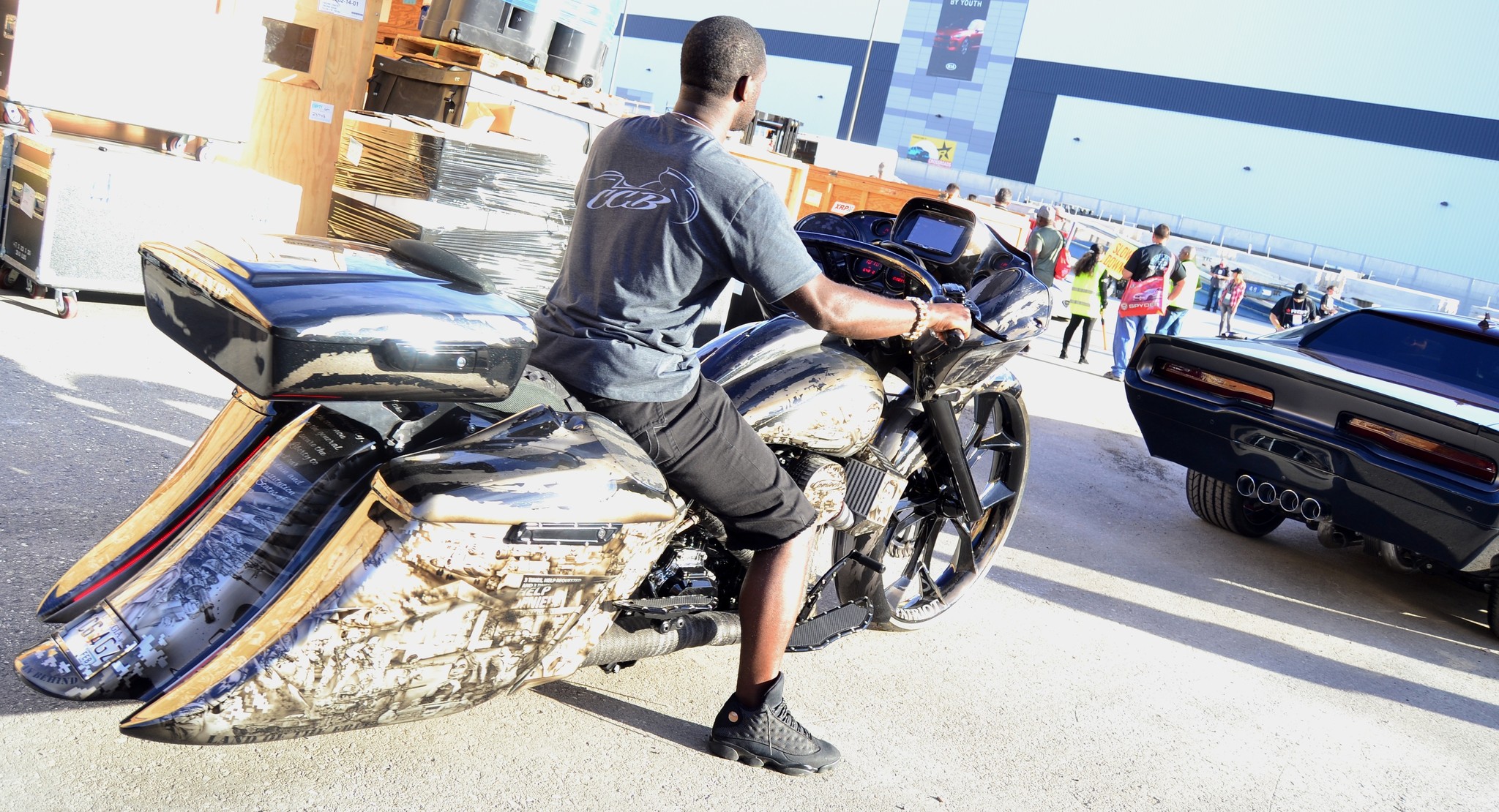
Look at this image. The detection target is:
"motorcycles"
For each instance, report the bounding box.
[13,194,1058,745]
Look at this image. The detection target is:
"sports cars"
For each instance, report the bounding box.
[1129,301,1498,639]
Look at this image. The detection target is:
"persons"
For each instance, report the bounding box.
[872,154,1499,386]
[526,14,973,780]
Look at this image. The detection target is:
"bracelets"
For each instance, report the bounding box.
[899,295,931,341]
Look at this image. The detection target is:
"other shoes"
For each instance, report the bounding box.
[1203,307,1217,312]
[1017,349,1029,355]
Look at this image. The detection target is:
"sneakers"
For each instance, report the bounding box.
[1059,354,1068,359]
[1104,370,1125,382]
[1078,358,1090,365]
[709,672,843,777]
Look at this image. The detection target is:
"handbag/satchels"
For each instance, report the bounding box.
[1054,247,1072,280]
[1117,274,1169,318]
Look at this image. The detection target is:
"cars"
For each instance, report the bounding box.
[934,18,986,54]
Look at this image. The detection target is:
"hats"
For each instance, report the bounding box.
[1053,206,1067,218]
[1231,268,1244,274]
[1292,283,1308,299]
[1034,204,1056,221]
